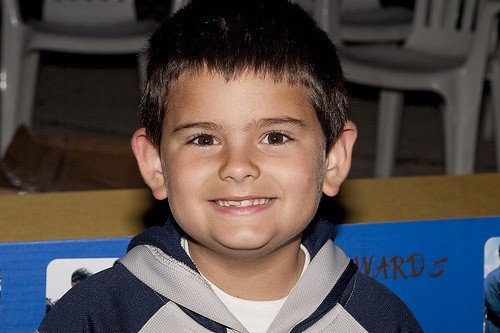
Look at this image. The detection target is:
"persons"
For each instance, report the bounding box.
[485,266,500,333]
[34,1,428,333]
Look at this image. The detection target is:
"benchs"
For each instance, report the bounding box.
[0,178,500,333]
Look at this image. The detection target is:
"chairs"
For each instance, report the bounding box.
[0,0,159,163]
[337,0,500,176]
